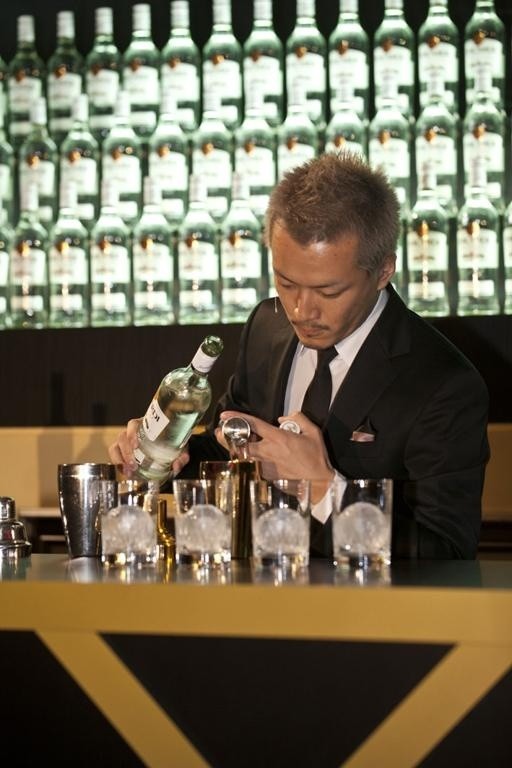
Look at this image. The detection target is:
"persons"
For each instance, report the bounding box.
[107,146,490,559]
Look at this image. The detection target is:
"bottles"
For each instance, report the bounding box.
[122,3,161,145]
[160,0,203,142]
[372,0,416,131]
[147,85,190,232]
[176,174,220,325]
[324,71,367,167]
[326,0,372,134]
[412,63,460,219]
[100,90,142,230]
[131,176,175,327]
[234,78,275,228]
[202,0,243,137]
[16,96,59,238]
[0,210,10,329]
[10,181,48,323]
[0,119,15,225]
[49,178,93,327]
[126,333,224,484]
[191,81,234,228]
[416,0,461,133]
[243,0,285,132]
[85,5,121,150]
[4,13,49,153]
[57,92,100,236]
[285,0,328,138]
[463,0,506,129]
[405,161,450,316]
[462,59,508,217]
[367,66,411,224]
[277,75,318,185]
[45,9,87,152]
[454,158,502,316]
[90,178,132,327]
[220,171,265,324]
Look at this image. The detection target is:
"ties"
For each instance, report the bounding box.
[298,339,338,427]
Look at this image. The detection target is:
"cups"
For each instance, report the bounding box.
[57,461,121,558]
[199,458,262,559]
[99,476,160,568]
[329,477,394,572]
[172,478,236,568]
[248,477,311,569]
